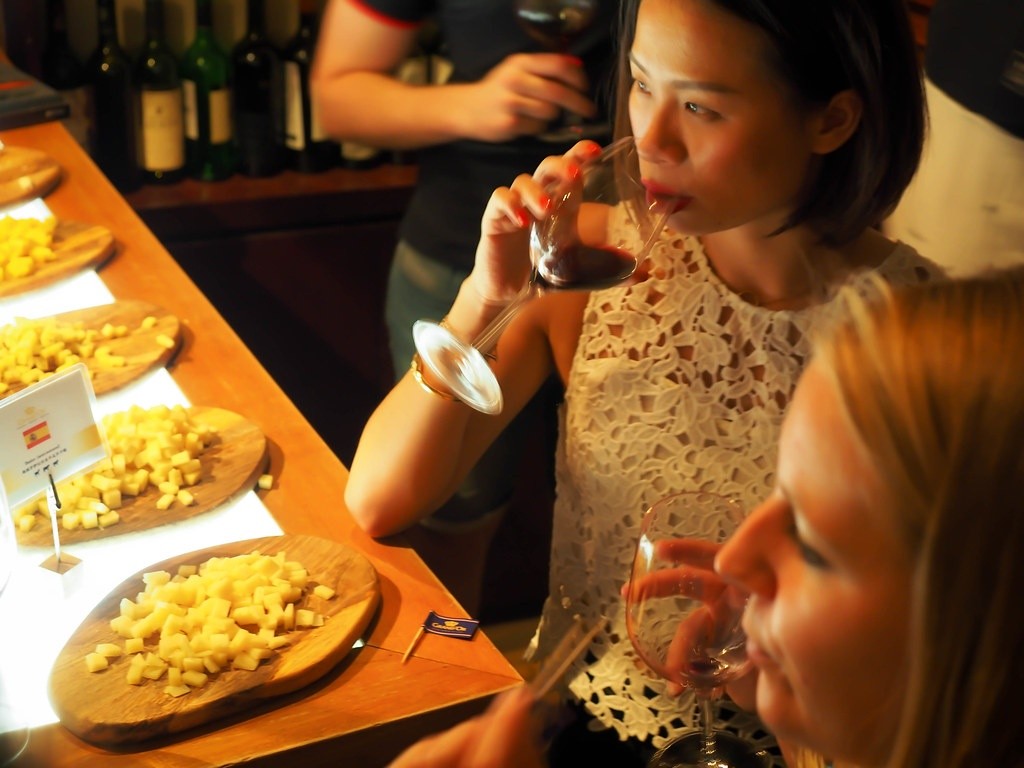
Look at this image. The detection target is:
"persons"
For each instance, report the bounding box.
[343,0,955,768]
[621,273,1024,768]
[310,0,633,629]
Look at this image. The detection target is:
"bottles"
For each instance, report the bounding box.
[4,0,454,185]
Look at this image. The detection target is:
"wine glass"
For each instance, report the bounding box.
[516,0,609,140]
[626,492,776,768]
[411,135,686,415]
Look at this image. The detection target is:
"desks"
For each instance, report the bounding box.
[0,120,526,768]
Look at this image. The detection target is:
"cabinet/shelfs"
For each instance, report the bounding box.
[118,163,420,473]
